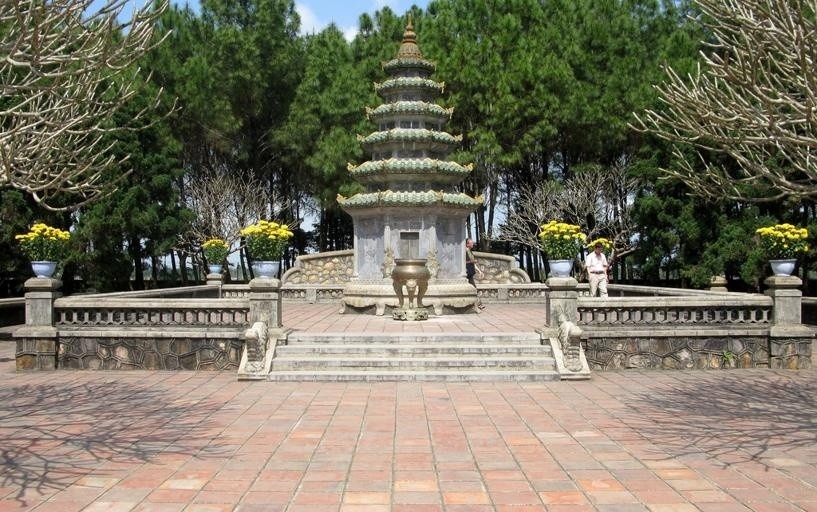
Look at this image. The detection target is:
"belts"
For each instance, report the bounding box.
[591,271,605,274]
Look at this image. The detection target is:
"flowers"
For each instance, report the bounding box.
[588,238,614,258]
[15,220,72,259]
[202,239,229,264]
[240,218,295,259]
[755,222,810,260]
[538,219,589,260]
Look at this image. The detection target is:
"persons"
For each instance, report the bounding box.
[582,242,609,297]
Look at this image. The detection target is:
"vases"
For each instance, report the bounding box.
[767,257,798,277]
[546,258,575,277]
[31,258,57,279]
[207,263,223,273]
[250,257,281,278]
